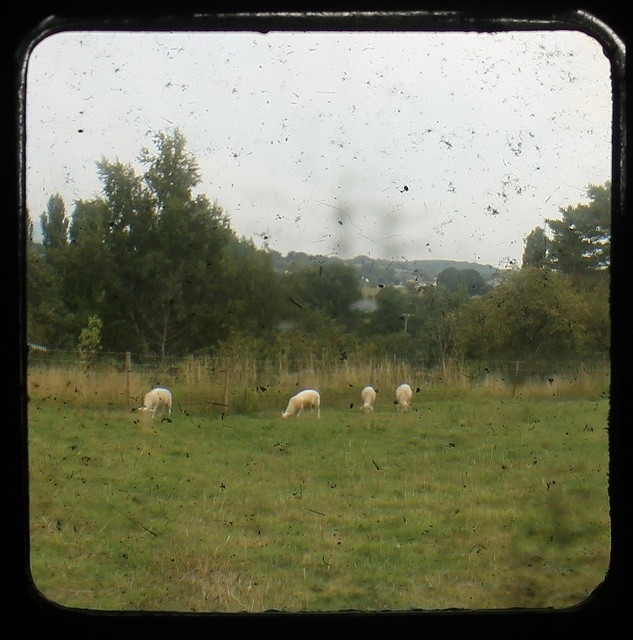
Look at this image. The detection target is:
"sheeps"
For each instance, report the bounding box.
[395,383,412,413]
[136,388,172,422]
[358,386,376,414]
[280,388,320,420]
